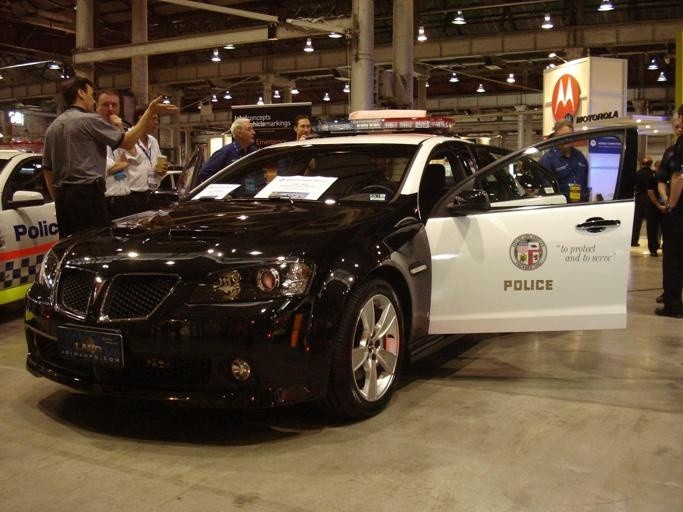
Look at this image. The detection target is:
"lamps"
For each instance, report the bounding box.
[416,10,557,95]
[162,31,351,106]
[46,62,74,80]
[598,0,614,11]
[647,59,667,84]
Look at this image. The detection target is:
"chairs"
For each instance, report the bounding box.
[377,164,446,207]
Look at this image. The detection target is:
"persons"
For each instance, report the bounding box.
[291,113,310,141]
[41,77,179,241]
[94,87,144,217]
[537,119,588,202]
[198,117,267,198]
[127,108,169,211]
[635,102,683,318]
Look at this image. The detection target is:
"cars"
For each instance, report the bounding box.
[0,152,61,310]
[23,118,639,418]
